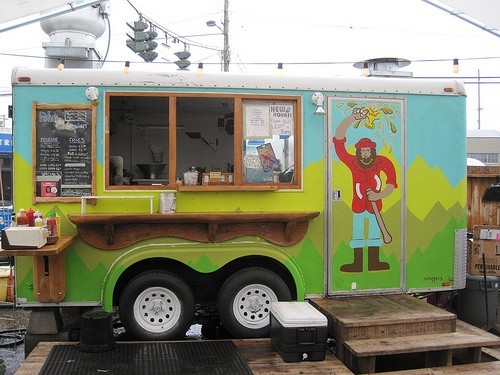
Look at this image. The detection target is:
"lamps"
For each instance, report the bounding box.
[312,92,326,114]
[85,87,100,106]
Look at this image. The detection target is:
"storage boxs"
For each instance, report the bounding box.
[269,301,329,362]
[5,226,47,248]
[0,266,15,302]
[201,172,234,185]
[468,225,500,275]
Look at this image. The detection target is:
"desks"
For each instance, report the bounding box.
[0,236,77,305]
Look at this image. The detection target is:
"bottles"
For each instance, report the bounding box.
[16,207,60,244]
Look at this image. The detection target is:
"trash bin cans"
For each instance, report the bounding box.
[0,328,25,375]
